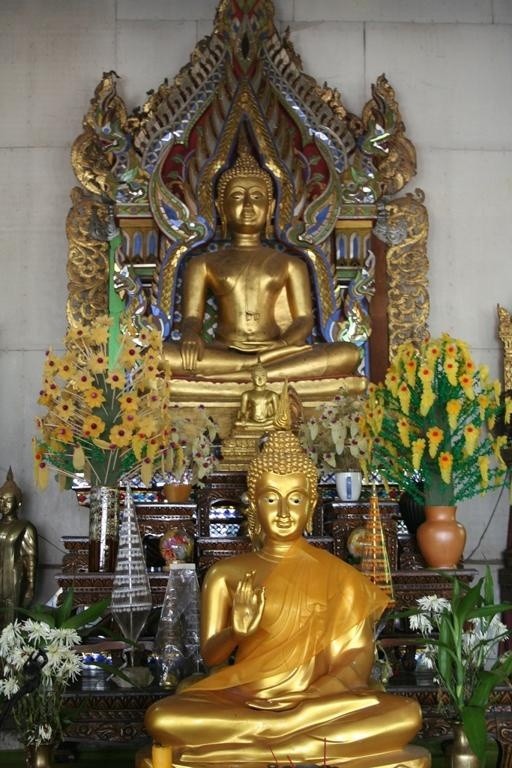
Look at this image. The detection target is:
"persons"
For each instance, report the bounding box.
[144,146,361,381]
[0,464,37,623]
[145,431,423,763]
[237,360,280,423]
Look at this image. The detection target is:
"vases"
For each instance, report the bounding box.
[335,471,362,502]
[25,744,55,768]
[452,727,481,768]
[88,484,119,572]
[166,485,191,503]
[417,504,466,569]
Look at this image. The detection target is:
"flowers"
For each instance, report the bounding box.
[31,314,174,491]
[141,404,220,489]
[359,332,512,506]
[299,387,370,472]
[0,588,142,749]
[374,552,512,767]
